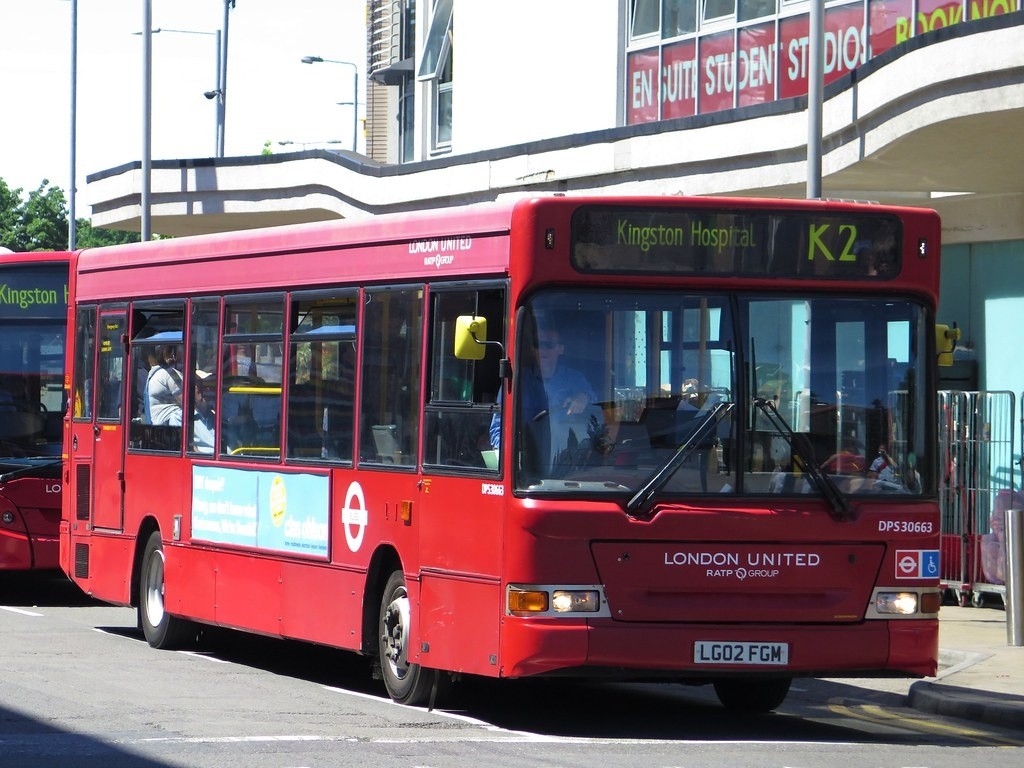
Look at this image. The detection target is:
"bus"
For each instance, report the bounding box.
[58,187,963,716]
[0,249,239,602]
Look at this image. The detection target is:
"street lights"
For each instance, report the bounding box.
[301,55,358,153]
[134,28,222,157]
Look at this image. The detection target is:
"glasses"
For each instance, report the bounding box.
[531,339,561,350]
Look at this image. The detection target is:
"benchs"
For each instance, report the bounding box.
[202,376,417,466]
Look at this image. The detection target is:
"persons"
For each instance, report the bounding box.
[117,342,276,453]
[681,379,716,491]
[489,322,604,478]
[869,445,922,493]
